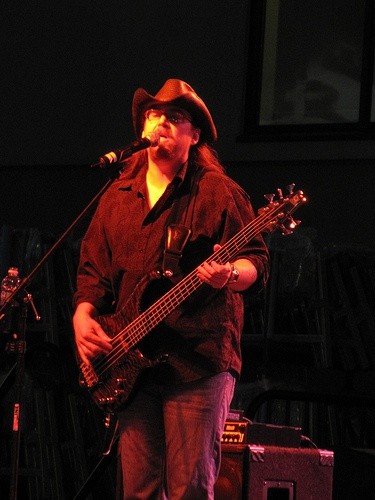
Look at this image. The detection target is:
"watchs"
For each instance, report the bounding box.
[228,262,239,288]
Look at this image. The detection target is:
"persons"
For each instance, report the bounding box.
[73,77,268,500]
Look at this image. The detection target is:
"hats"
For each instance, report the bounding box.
[131,78,218,144]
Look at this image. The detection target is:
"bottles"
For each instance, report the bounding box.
[1,267,20,306]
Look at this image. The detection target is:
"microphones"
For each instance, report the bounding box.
[99,133,160,168]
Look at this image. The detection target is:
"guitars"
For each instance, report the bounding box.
[73,181,308,413]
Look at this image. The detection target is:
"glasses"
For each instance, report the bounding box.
[142,108,196,124]
